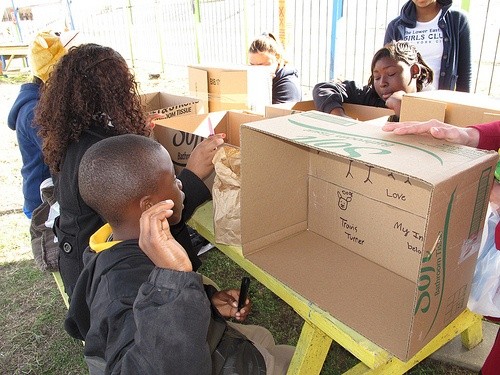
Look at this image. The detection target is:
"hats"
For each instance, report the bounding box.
[56,29,85,51]
[28,31,69,83]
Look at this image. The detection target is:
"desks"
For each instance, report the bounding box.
[186,199,484,375]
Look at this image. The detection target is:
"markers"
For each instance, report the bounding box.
[208,117,216,136]
[238,277,250,313]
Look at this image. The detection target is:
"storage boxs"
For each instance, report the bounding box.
[134,64,500,363]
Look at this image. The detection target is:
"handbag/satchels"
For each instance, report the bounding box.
[29,186,61,274]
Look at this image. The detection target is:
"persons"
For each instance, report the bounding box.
[382,118,500,375]
[312,39,434,123]
[64,133,298,375]
[7,32,226,305]
[384,0,472,93]
[248,32,299,104]
